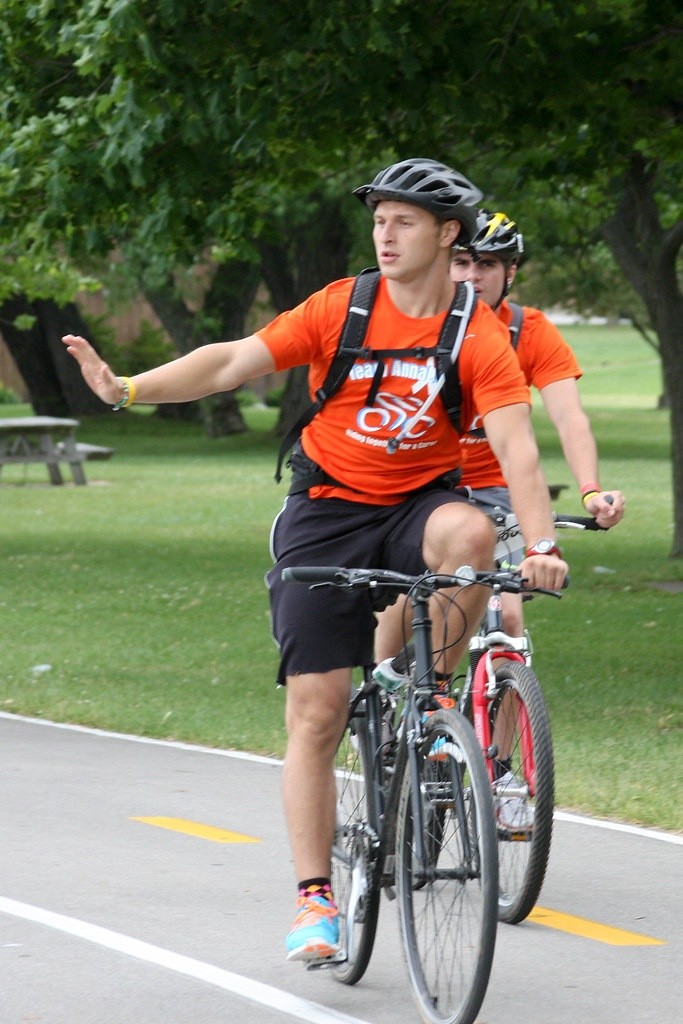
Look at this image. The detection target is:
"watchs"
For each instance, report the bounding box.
[525,537,563,559]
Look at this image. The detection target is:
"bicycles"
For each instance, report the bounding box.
[282,564,569,1023]
[377,493,615,925]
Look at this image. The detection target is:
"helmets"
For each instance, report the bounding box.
[352,157,483,246]
[450,209,525,270]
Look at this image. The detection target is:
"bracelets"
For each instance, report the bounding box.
[111,376,129,412]
[121,377,135,410]
[581,483,600,506]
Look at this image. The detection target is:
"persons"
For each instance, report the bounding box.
[348,209,629,831]
[60,157,568,963]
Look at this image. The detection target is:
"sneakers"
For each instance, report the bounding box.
[287,896,343,962]
[491,770,536,833]
